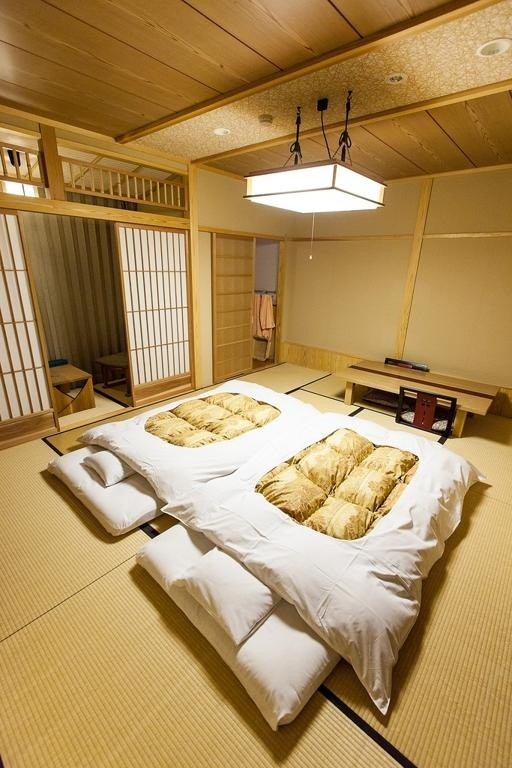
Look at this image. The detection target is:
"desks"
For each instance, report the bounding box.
[331,359,500,438]
[94,353,131,397]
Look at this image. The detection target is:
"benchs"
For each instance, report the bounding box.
[50,363,96,419]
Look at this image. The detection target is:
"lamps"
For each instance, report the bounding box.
[240,88,387,214]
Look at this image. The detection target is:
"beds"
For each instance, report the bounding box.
[43,378,482,732]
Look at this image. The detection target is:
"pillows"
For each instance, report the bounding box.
[80,446,134,488]
[171,547,285,644]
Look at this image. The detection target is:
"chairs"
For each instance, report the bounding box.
[395,384,457,438]
